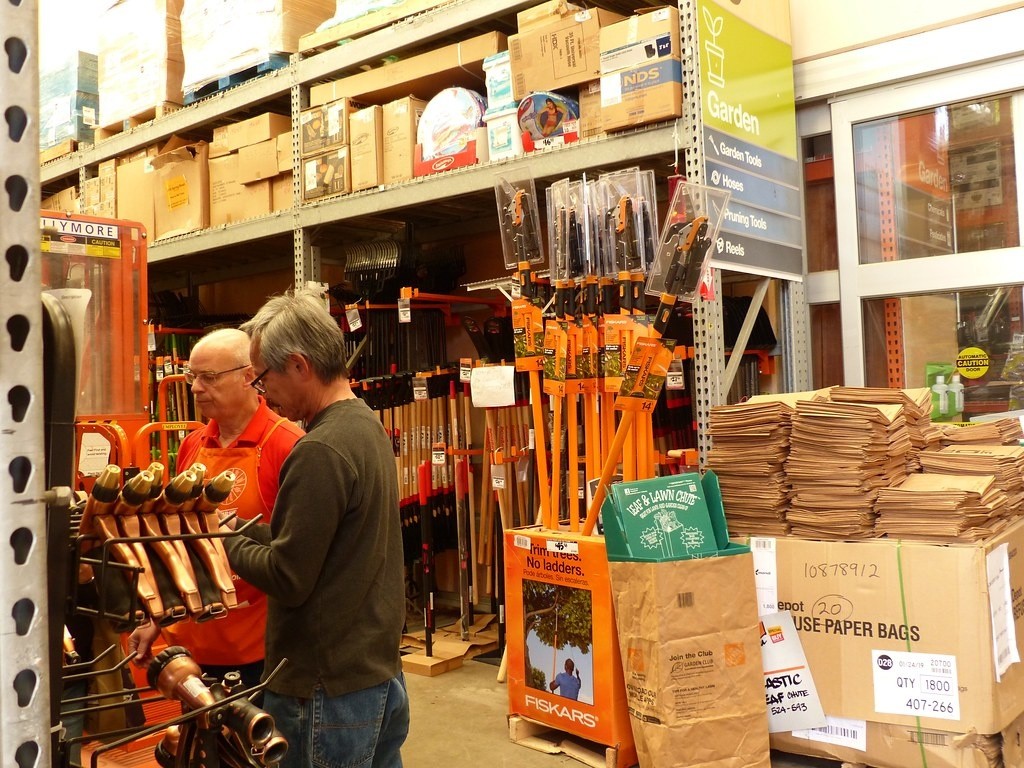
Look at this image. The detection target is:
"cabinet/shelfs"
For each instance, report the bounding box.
[802,108,962,390]
[948,92,1024,423]
[41,1,816,486]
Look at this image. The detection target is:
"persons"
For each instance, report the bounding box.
[179,328,310,723]
[536,98,567,137]
[549,658,581,702]
[216,287,408,768]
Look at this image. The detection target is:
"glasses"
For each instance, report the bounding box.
[182,364,249,386]
[249,365,273,393]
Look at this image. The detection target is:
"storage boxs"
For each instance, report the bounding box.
[39,1,685,249]
[503,525,641,768]
